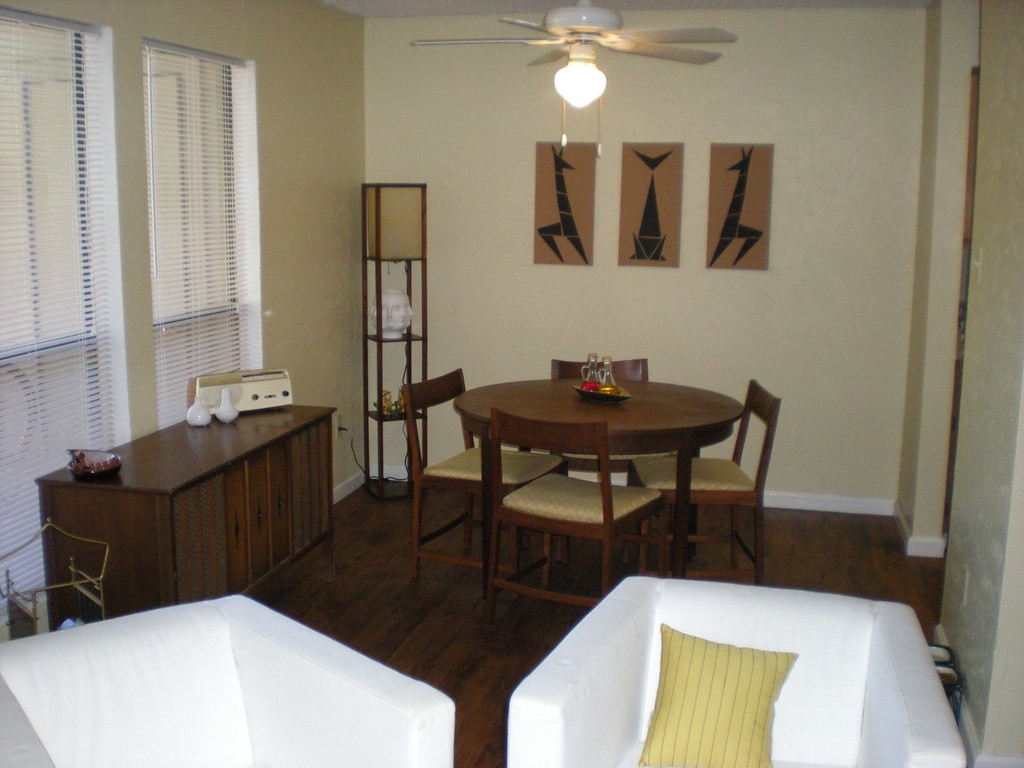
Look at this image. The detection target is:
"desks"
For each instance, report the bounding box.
[453,379,745,601]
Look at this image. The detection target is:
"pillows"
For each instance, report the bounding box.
[636,623,799,768]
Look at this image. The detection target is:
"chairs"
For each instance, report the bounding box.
[628,379,782,587]
[481,406,668,626]
[402,368,566,602]
[550,358,649,477]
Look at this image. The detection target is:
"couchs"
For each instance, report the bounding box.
[0,594,456,768]
[506,577,970,768]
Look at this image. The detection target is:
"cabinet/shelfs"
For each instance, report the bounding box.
[362,182,429,502]
[34,405,337,632]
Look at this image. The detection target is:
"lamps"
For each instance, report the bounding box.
[552,44,605,108]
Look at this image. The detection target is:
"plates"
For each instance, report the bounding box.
[573,384,632,403]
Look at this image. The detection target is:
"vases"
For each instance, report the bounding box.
[186,395,211,427]
[215,387,240,424]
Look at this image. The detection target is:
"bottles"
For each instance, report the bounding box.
[187,397,212,427]
[581,353,600,392]
[214,388,239,424]
[597,356,620,395]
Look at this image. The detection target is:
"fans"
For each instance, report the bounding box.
[410,0,738,157]
